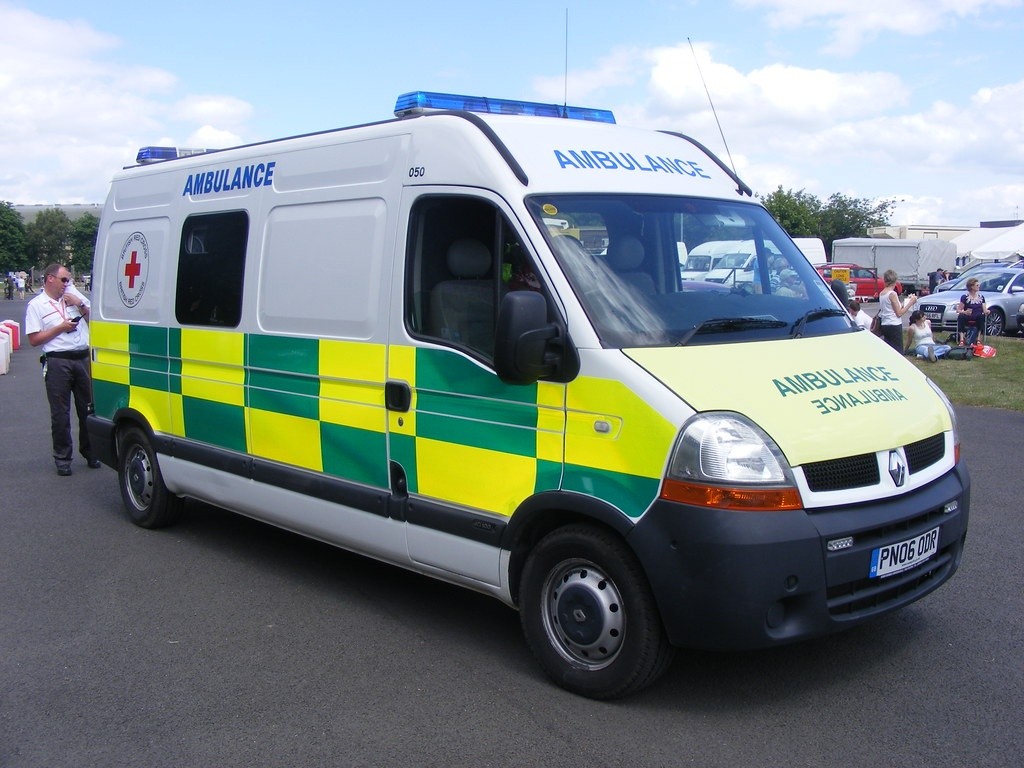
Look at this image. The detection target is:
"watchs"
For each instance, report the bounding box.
[77,302,85,308]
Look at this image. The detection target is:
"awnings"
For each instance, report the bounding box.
[949,227,1016,260]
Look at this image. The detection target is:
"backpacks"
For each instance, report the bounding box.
[945,346,972,360]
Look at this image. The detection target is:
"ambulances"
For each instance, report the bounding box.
[84,92,971,701]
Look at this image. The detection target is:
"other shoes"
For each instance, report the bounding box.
[57,465,71,476]
[928,346,937,362]
[958,340,966,346]
[976,340,981,345]
[87,458,101,468]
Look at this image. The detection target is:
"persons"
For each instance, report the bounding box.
[903,310,952,363]
[25,264,101,475]
[753,248,810,300]
[3,273,36,300]
[932,268,951,292]
[847,298,874,330]
[878,269,918,355]
[957,279,991,347]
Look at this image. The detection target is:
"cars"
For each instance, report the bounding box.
[912,267,1024,337]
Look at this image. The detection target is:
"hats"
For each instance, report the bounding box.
[937,268,943,271]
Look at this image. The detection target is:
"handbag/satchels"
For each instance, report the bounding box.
[974,344,997,358]
[870,315,882,336]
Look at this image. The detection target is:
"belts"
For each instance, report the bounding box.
[45,349,89,359]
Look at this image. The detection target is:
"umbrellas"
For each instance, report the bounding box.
[971,221,1024,260]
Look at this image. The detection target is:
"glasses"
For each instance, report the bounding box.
[49,274,69,283]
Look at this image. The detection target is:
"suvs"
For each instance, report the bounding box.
[933,260,1024,294]
[812,262,902,301]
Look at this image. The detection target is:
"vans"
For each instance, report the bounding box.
[680,238,746,282]
[704,238,828,287]
[598,241,689,268]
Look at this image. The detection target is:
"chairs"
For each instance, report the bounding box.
[956,311,987,344]
[428,240,509,352]
[608,233,658,300]
